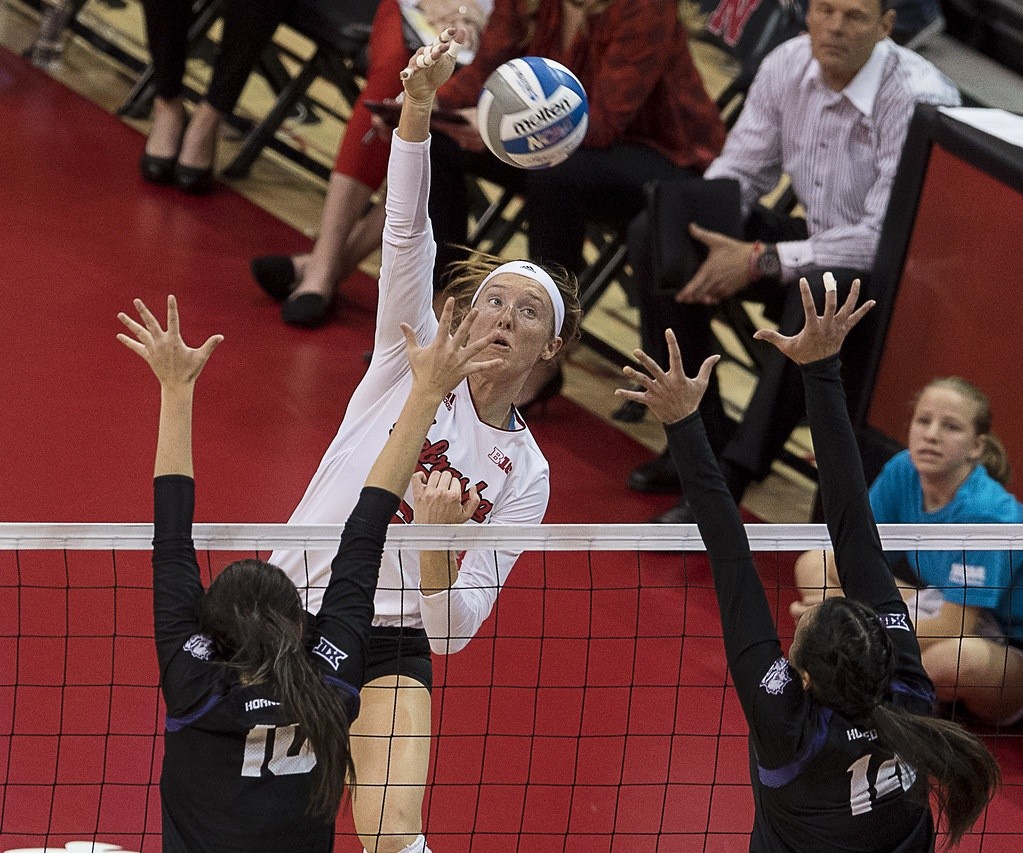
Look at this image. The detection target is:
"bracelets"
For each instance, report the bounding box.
[749,240,760,284]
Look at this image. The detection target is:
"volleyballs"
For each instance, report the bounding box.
[476,57,588,169]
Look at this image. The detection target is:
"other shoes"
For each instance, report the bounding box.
[281,291,332,329]
[612,384,648,422]
[251,253,297,303]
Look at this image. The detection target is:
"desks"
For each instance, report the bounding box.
[810,102,1023,523]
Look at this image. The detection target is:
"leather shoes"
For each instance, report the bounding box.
[648,504,696,524]
[629,449,681,494]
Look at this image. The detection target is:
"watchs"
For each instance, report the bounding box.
[756,243,781,279]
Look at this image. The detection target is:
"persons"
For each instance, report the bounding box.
[0,0,1023,853]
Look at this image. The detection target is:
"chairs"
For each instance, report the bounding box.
[24,0,800,375]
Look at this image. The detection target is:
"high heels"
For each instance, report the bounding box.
[139,106,188,185]
[174,132,218,193]
[517,368,563,415]
